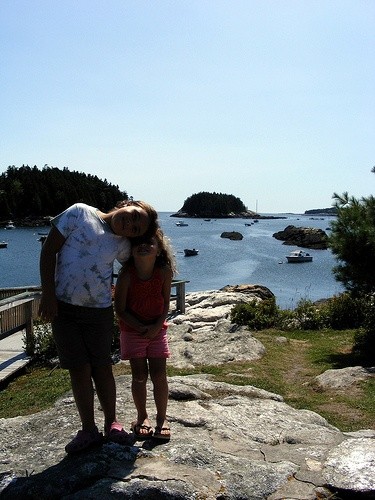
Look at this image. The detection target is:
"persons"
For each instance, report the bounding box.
[112,225,171,442]
[36,200,159,455]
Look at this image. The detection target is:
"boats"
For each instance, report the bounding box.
[175,220,190,226]
[183,248,199,257]
[285,250,314,263]
[6,221,16,231]
[0,241,9,248]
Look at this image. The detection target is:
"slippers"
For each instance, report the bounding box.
[65,430,102,452]
[131,422,154,439]
[104,422,131,445]
[155,426,171,441]
